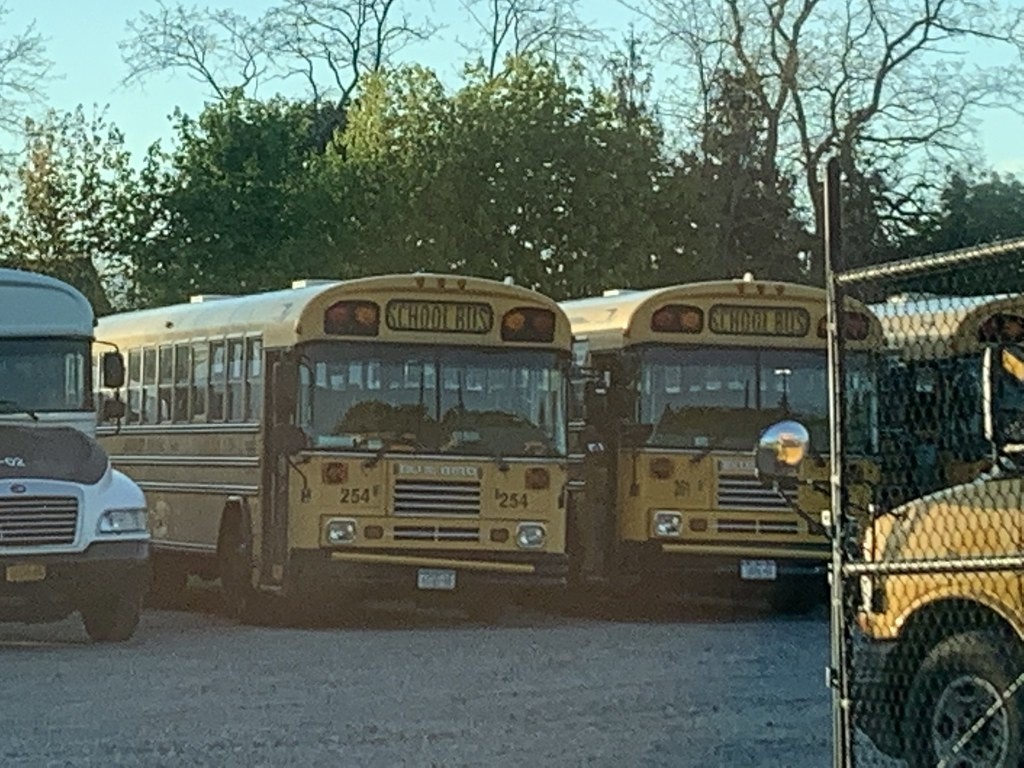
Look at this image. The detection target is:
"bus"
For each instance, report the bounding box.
[858,283,1023,490]
[79,269,579,614]
[538,273,887,617]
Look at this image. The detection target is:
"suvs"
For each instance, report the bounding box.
[744,417,1023,768]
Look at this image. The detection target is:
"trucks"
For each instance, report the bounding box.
[0,262,159,646]
